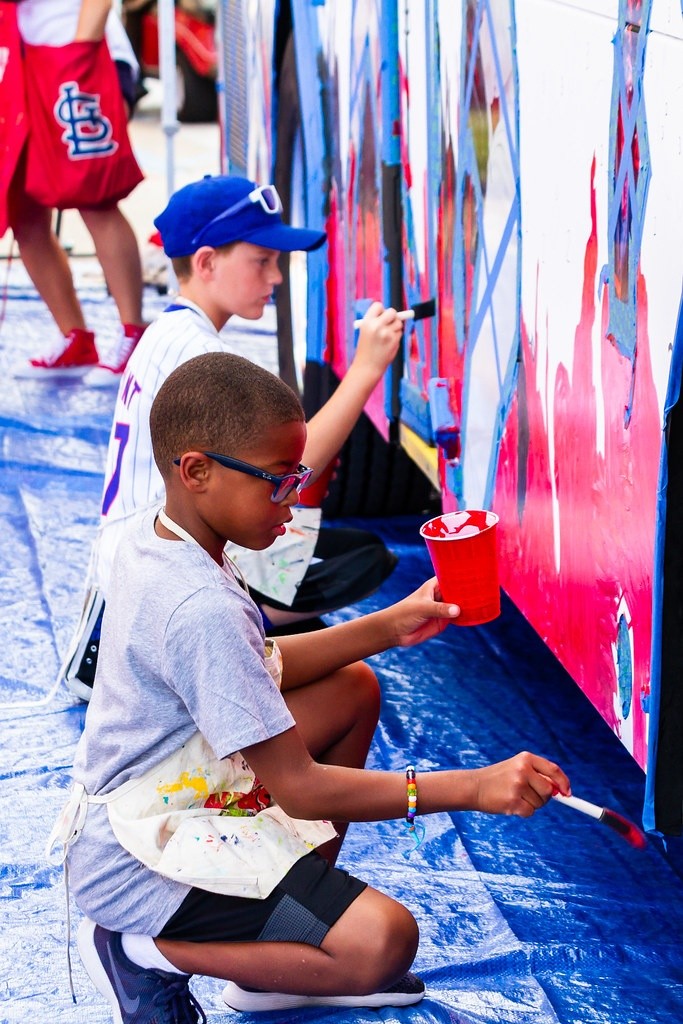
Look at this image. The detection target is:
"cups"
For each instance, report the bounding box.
[420,511,501,626]
[298,458,335,507]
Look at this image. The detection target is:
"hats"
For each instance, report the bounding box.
[149,175,327,258]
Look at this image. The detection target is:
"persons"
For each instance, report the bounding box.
[0,0,409,708]
[60,352,571,1024]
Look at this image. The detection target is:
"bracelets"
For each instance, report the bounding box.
[404,767,419,824]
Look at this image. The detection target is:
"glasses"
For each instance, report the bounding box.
[192,183,283,243]
[173,451,314,504]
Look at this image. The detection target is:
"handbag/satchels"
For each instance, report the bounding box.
[23,39,144,210]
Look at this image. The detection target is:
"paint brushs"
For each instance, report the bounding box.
[353,299,437,332]
[550,785,646,848]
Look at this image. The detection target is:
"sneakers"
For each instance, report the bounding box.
[222,971,426,1012]
[64,591,106,702]
[83,323,149,388]
[76,917,207,1024]
[9,328,100,378]
